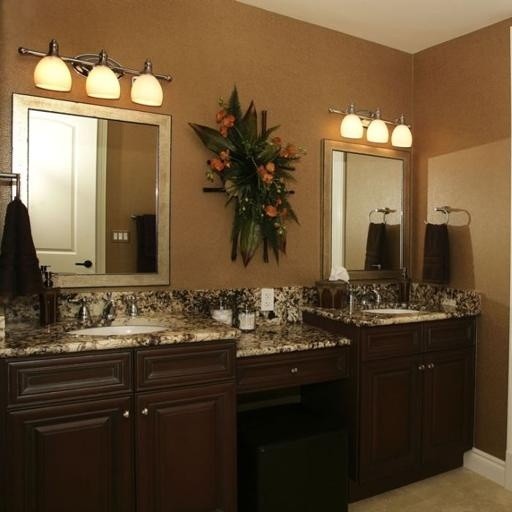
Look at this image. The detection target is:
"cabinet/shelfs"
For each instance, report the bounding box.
[0,339,235,512]
[304,313,473,503]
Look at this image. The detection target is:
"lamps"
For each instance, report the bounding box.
[329,104,413,148]
[19,40,172,107]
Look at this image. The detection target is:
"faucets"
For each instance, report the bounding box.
[368,289,382,304]
[102,292,118,322]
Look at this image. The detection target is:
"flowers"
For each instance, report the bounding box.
[189,81,306,265]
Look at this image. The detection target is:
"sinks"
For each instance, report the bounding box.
[64,317,170,335]
[359,304,430,314]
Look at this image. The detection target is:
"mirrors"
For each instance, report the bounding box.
[321,138,409,275]
[7,92,173,287]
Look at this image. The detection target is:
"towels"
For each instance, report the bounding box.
[365,220,387,272]
[0,196,43,298]
[136,214,156,274]
[423,224,452,285]
